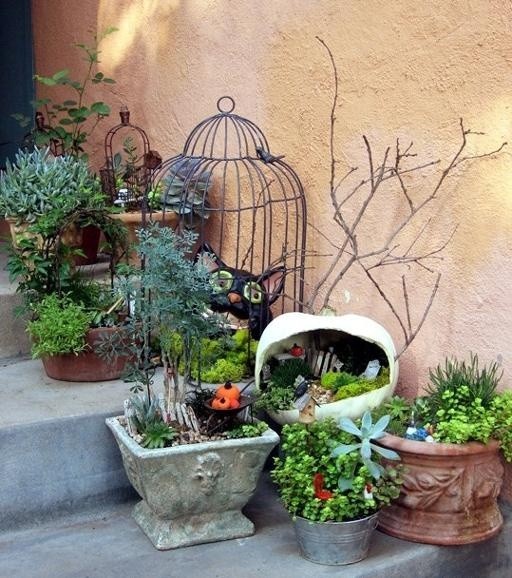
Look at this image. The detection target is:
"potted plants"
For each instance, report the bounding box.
[255,331,391,422]
[98,101,181,270]
[2,26,119,269]
[270,416,410,567]
[105,227,281,550]
[372,352,512,548]
[0,145,145,383]
